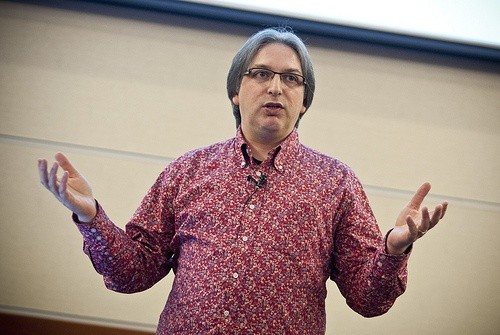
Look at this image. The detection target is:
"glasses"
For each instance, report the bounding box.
[242,67,307,87]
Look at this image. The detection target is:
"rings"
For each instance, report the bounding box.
[416,225,427,235]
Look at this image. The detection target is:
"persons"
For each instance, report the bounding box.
[40,23,448,334]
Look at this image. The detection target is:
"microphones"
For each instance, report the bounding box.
[247,174,267,189]
